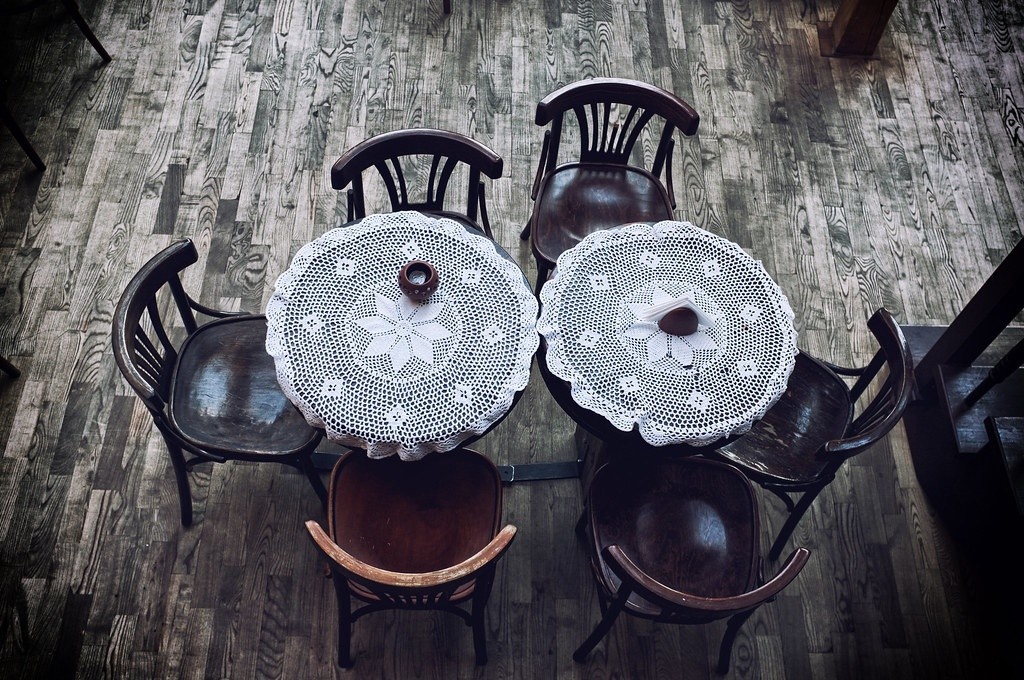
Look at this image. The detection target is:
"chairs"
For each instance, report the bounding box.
[112,237,330,528]
[713,306,917,560]
[305,443,518,668]
[521,77,701,310]
[330,126,505,241]
[572,450,813,680]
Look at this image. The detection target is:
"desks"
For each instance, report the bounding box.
[539,216,799,451]
[264,207,542,462]
